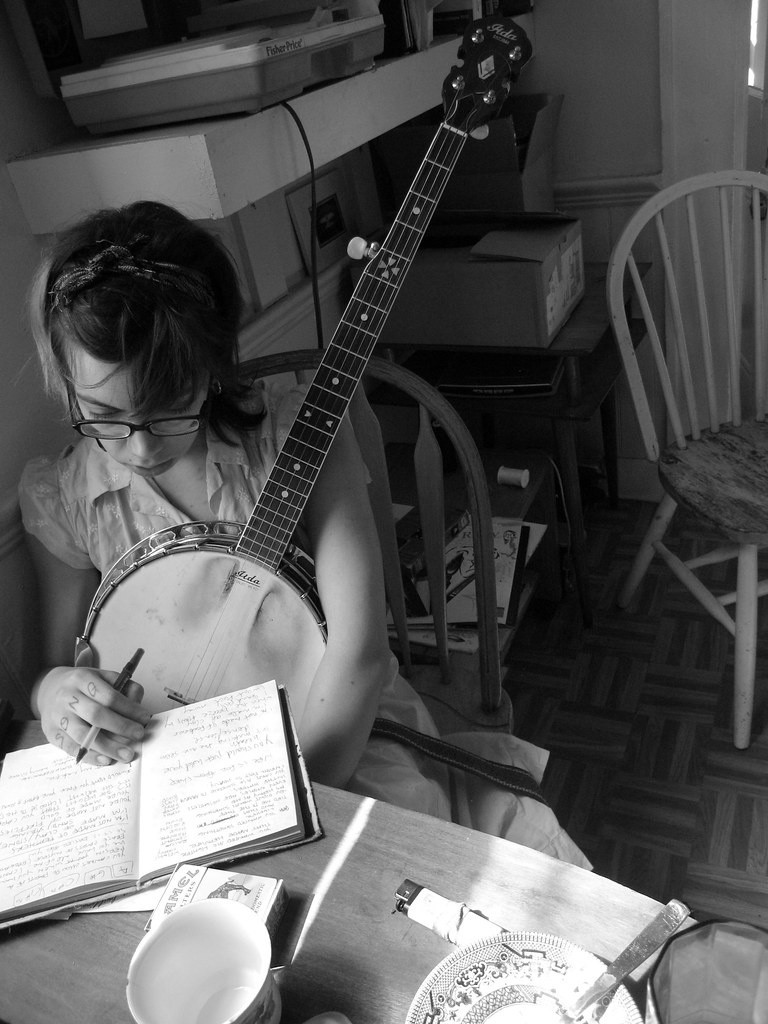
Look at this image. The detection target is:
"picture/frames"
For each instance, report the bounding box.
[286,168,357,277]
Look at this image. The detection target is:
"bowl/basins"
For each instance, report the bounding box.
[126,898,281,1024]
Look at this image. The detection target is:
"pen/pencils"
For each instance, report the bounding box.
[75,647,146,764]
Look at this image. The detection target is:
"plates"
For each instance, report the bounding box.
[405,931,643,1024]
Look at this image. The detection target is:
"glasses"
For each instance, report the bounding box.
[67,381,213,440]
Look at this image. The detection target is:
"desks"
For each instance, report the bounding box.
[362,261,652,695]
[0,720,699,1024]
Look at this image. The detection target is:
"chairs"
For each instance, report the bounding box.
[237,346,515,736]
[606,169,768,750]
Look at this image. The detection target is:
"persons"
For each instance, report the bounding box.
[16,200,451,821]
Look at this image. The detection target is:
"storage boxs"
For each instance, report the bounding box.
[345,208,586,349]
[377,94,565,213]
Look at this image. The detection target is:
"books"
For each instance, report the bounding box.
[0,676,325,934]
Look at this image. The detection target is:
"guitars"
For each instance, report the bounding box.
[74,17,530,751]
